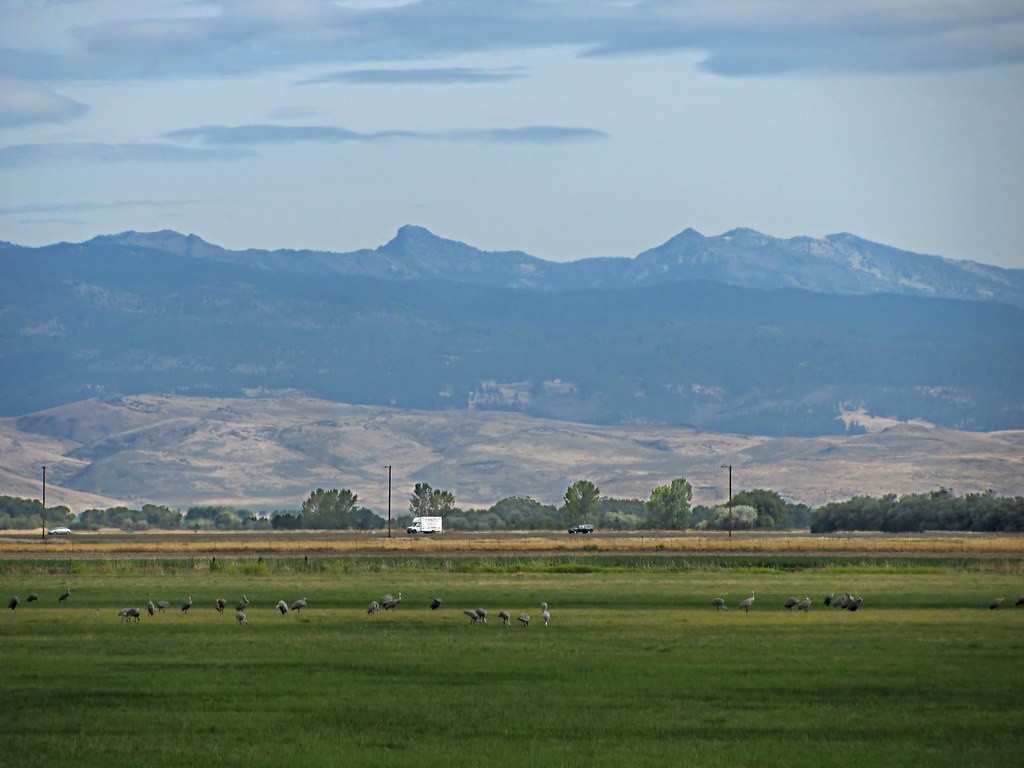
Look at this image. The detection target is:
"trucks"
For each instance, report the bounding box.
[406,516,443,534]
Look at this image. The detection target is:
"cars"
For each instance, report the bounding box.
[47,527,71,536]
[569,524,594,534]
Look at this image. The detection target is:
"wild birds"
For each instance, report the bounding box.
[117,608,140,625]
[463,608,487,624]
[145,592,155,617]
[26,592,38,607]
[498,611,512,627]
[1015,596,1024,607]
[215,598,225,613]
[276,599,288,615]
[517,614,530,627]
[738,590,755,614]
[8,595,20,610]
[823,591,863,613]
[380,592,401,613]
[710,598,728,612]
[784,596,811,614]
[430,597,442,610]
[540,602,550,628]
[291,596,307,614]
[157,600,169,614]
[234,595,249,625]
[182,596,192,616]
[367,601,379,616]
[58,589,71,603]
[990,593,1005,610]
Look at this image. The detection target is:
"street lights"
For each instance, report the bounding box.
[721,464,732,538]
[385,465,392,538]
